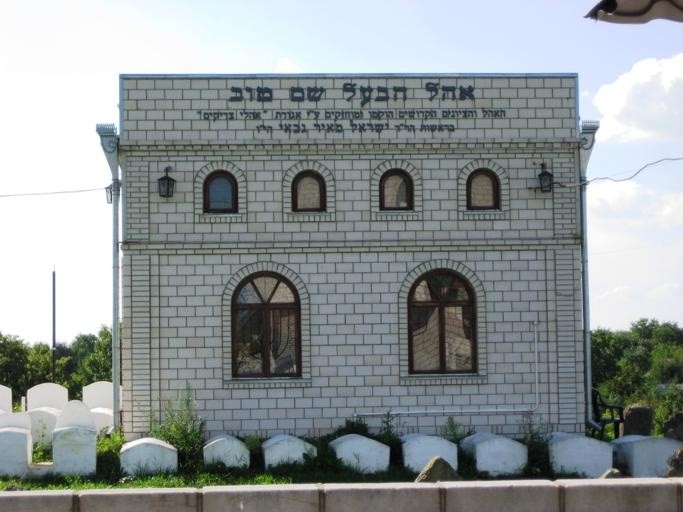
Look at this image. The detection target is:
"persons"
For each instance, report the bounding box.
[463,317,473,336]
[250,334,261,353]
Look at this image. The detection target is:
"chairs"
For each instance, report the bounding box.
[591,388,623,439]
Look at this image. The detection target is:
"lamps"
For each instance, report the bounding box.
[158,167,175,198]
[536,163,555,194]
